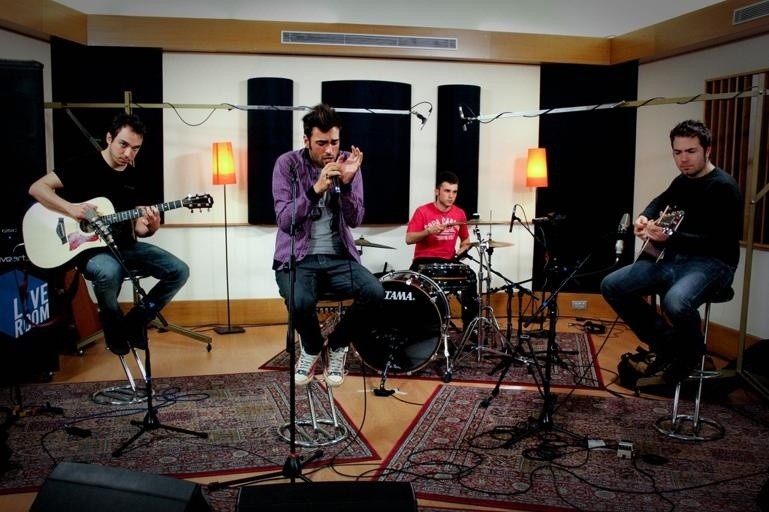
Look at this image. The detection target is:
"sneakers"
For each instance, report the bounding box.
[99,302,149,356]
[640,354,716,383]
[290,339,323,385]
[323,338,349,386]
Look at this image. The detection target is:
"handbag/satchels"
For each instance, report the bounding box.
[618,349,718,400]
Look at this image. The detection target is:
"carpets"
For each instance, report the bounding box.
[369,385,769,512]
[259,328,609,392]
[198,484,242,512]
[0,371,382,495]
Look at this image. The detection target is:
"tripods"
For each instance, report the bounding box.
[110,289,209,456]
[458,105,468,132]
[449,235,587,450]
[205,163,325,492]
[419,107,432,131]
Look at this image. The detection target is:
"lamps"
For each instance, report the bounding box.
[211,144,246,335]
[519,147,549,324]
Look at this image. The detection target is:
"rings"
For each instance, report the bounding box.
[326,174,329,180]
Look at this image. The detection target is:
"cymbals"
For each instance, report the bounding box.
[354,240,395,248]
[444,219,503,225]
[462,241,515,247]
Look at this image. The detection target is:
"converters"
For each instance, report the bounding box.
[70,424,92,436]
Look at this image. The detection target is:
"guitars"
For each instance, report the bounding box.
[23,193,214,272]
[633,206,684,263]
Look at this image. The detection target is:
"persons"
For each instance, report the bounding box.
[405,172,488,346]
[600,120,745,384]
[271,104,387,387]
[28,113,191,356]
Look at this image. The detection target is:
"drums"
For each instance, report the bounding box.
[419,264,471,291]
[350,270,450,376]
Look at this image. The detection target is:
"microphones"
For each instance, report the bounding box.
[332,174,341,195]
[85,208,118,249]
[614,212,631,262]
[509,204,516,233]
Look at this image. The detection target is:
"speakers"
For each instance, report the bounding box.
[28,460,213,512]
[234,481,418,512]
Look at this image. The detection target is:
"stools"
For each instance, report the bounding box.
[654,285,734,446]
[277,299,354,447]
[67,264,155,405]
